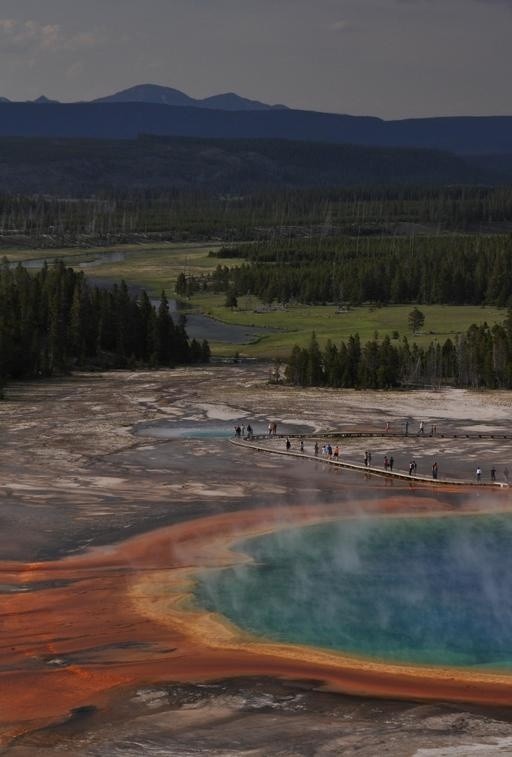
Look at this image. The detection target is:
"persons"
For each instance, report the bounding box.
[313,442,339,460]
[476,466,481,482]
[300,440,305,452]
[368,452,374,468]
[502,465,509,481]
[383,456,388,471]
[405,421,410,433]
[417,420,425,433]
[363,452,368,466]
[285,438,291,450]
[385,421,390,432]
[408,462,414,475]
[232,420,278,442]
[489,467,496,481]
[412,460,418,476]
[432,462,439,479]
[388,456,394,471]
